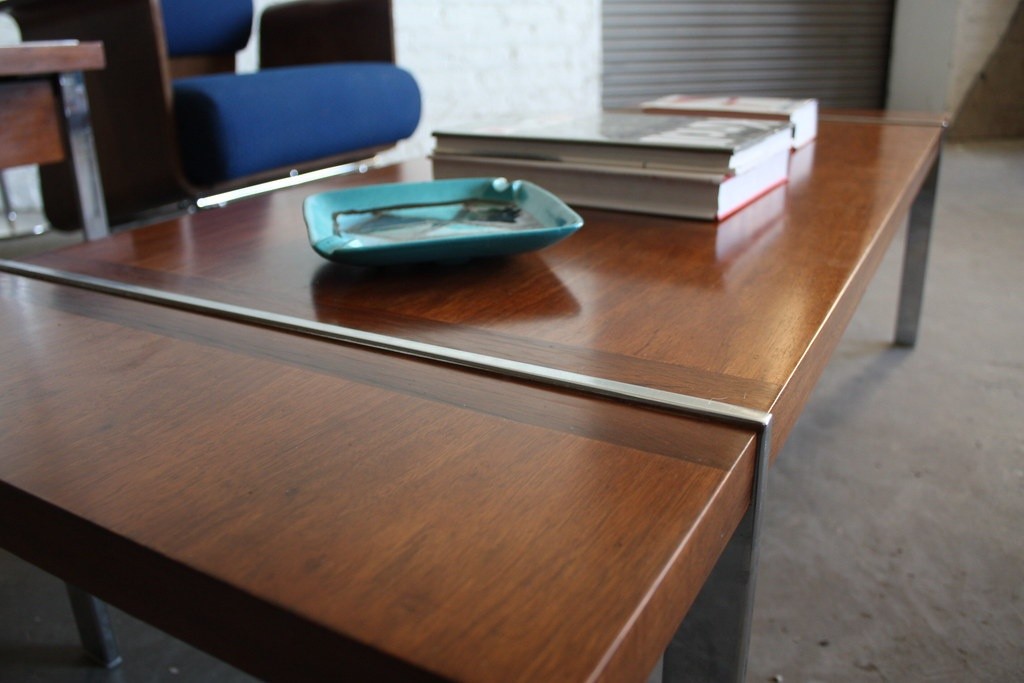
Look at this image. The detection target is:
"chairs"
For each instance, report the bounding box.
[10,0,422,233]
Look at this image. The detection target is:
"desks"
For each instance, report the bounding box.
[0,106,955,683]
[0,39,110,245]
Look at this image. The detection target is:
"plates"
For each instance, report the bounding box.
[301,176,583,264]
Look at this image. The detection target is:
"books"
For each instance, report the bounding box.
[432,93,820,226]
